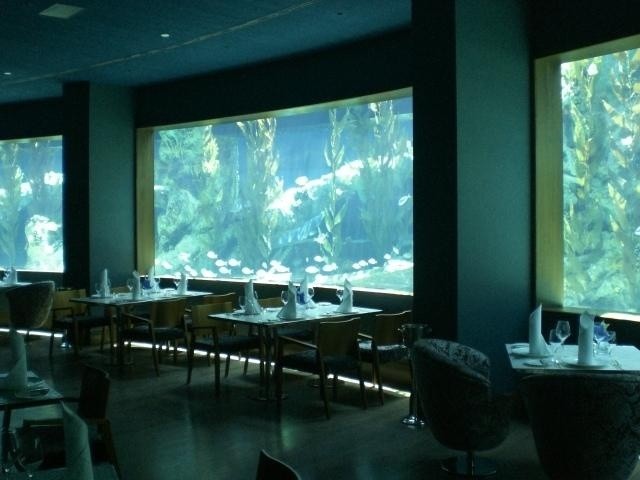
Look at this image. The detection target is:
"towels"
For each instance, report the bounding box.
[578,311,594,365]
[528,303,549,354]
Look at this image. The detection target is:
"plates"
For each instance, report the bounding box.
[563,357,613,366]
[512,347,554,356]
[522,360,559,366]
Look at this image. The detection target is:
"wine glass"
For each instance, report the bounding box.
[307,287,314,298]
[1,426,24,480]
[4,266,11,277]
[336,288,343,302]
[593,322,606,351]
[549,329,561,355]
[173,277,180,287]
[556,320,570,350]
[12,430,45,479]
[126,279,133,291]
[281,291,288,305]
[95,283,101,295]
[239,296,246,310]
[154,276,160,285]
